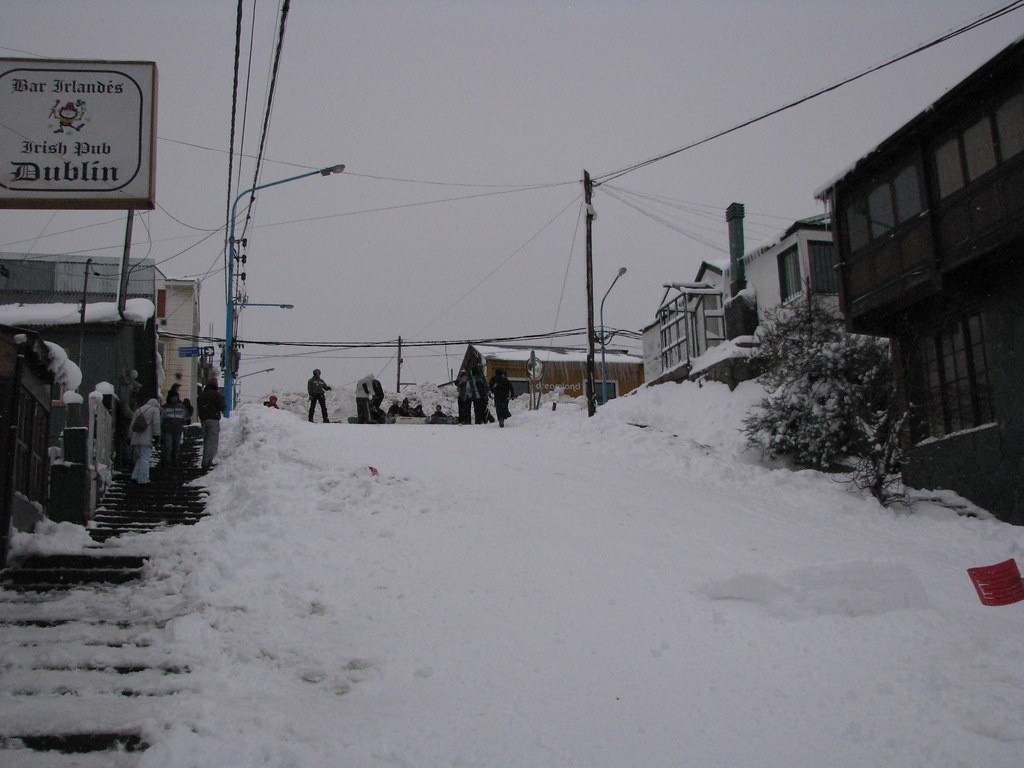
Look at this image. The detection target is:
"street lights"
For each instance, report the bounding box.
[221,163,347,418]
[600,266,627,405]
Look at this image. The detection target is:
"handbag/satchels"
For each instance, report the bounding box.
[464,370,481,401]
[363,383,368,393]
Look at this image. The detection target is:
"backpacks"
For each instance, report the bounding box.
[132,407,153,433]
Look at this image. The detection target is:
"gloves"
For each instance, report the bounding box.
[155,436,159,445]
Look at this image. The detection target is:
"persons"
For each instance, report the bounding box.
[161,383,193,458]
[268,396,279,409]
[129,398,161,483]
[197,378,226,468]
[388,397,426,417]
[356,374,384,424]
[308,369,331,423]
[431,405,447,417]
[455,365,514,428]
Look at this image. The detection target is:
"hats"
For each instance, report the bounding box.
[403,397,408,405]
[209,378,218,386]
